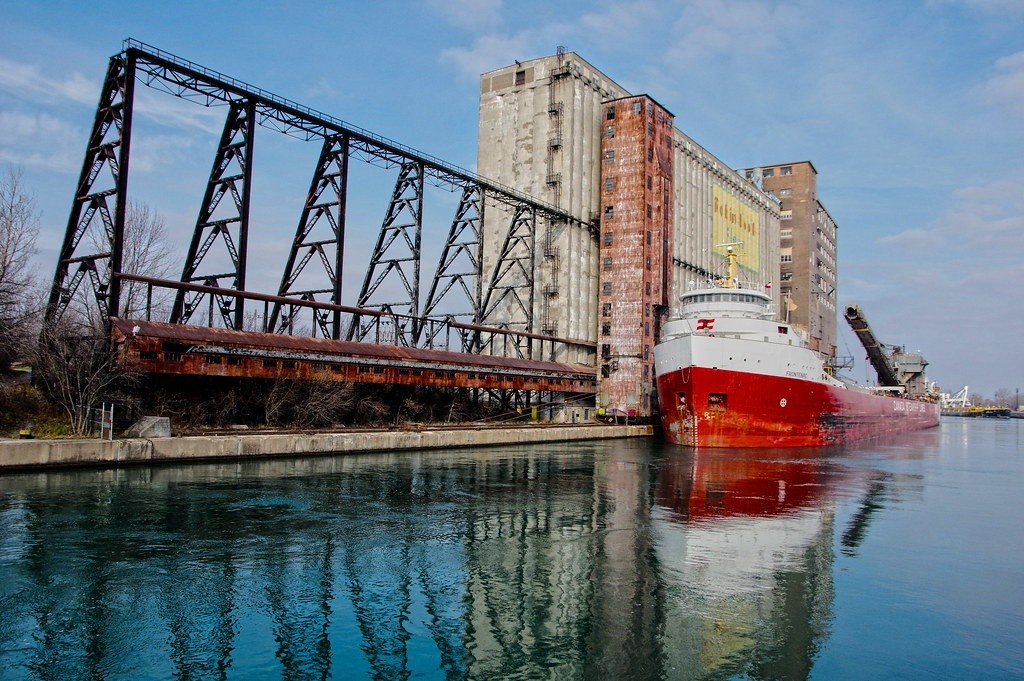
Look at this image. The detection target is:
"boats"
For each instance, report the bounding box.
[942,386,976,417]
[654,242,942,448]
[981,408,1012,419]
